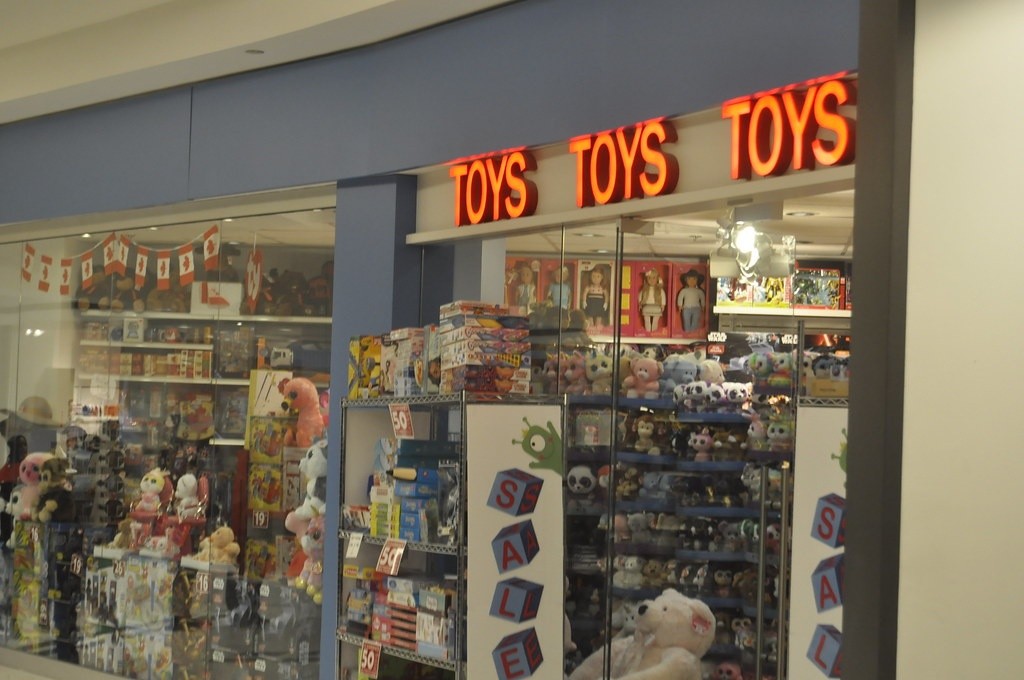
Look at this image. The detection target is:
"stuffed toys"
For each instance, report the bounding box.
[0,378,328,605]
[71,265,185,314]
[531,309,849,680]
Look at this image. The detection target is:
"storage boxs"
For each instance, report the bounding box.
[190,281,245,314]
[77,321,327,516]
[809,378,848,398]
[343,436,460,543]
[716,267,853,312]
[347,298,532,396]
[344,566,454,658]
[506,255,711,339]
[13,521,320,680]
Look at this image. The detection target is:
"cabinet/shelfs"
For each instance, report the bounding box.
[342,395,567,680]
[522,312,854,680]
[69,307,332,451]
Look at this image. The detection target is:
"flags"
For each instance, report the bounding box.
[81,252,93,289]
[102,233,118,276]
[114,235,129,277]
[204,227,220,271]
[60,258,72,295]
[38,255,52,292]
[135,247,149,291]
[156,252,170,290]
[178,245,194,286]
[22,243,36,282]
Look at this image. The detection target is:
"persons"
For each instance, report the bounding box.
[638,269,666,332]
[547,267,572,309]
[507,261,540,315]
[583,268,608,329]
[677,270,705,332]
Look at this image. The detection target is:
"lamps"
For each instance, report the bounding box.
[716,222,757,254]
